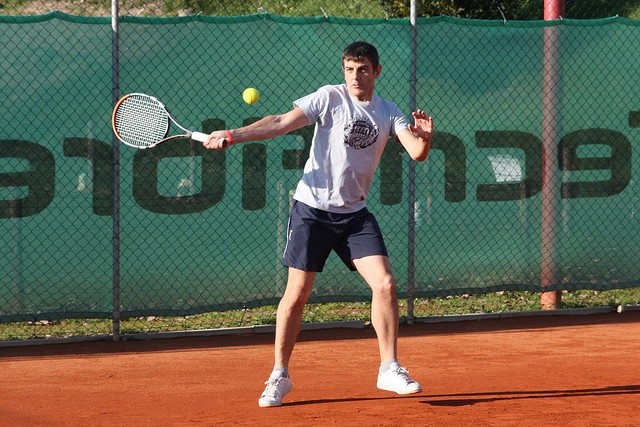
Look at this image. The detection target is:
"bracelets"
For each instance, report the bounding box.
[226,129,234,145]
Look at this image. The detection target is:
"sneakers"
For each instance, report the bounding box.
[258,371,291,407]
[377,362,422,395]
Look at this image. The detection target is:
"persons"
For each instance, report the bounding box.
[203,42,433,408]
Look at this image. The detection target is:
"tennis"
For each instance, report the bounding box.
[243,88,259,103]
